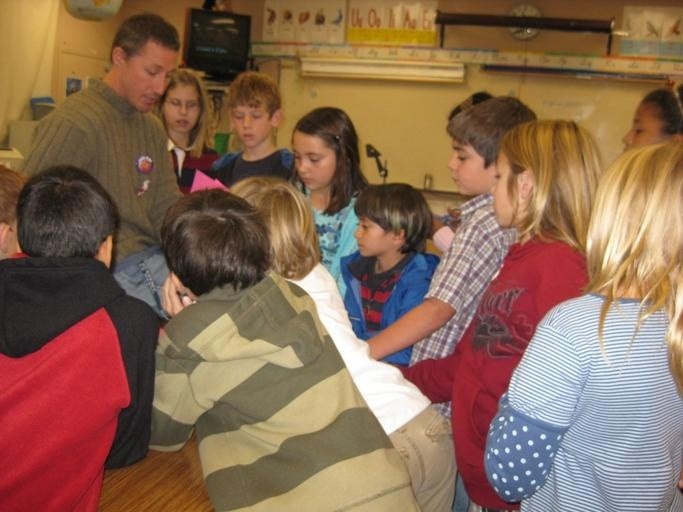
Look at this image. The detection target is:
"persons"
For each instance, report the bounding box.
[152,68,219,197]
[209,70,295,188]
[0,165,25,261]
[0,165,161,512]
[18,12,186,321]
[339,91,537,424]
[285,106,371,303]
[148,187,423,512]
[394,122,604,512]
[620,83,682,152]
[482,138,682,512]
[230,175,459,512]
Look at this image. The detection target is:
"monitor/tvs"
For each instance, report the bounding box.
[182,8,251,82]
